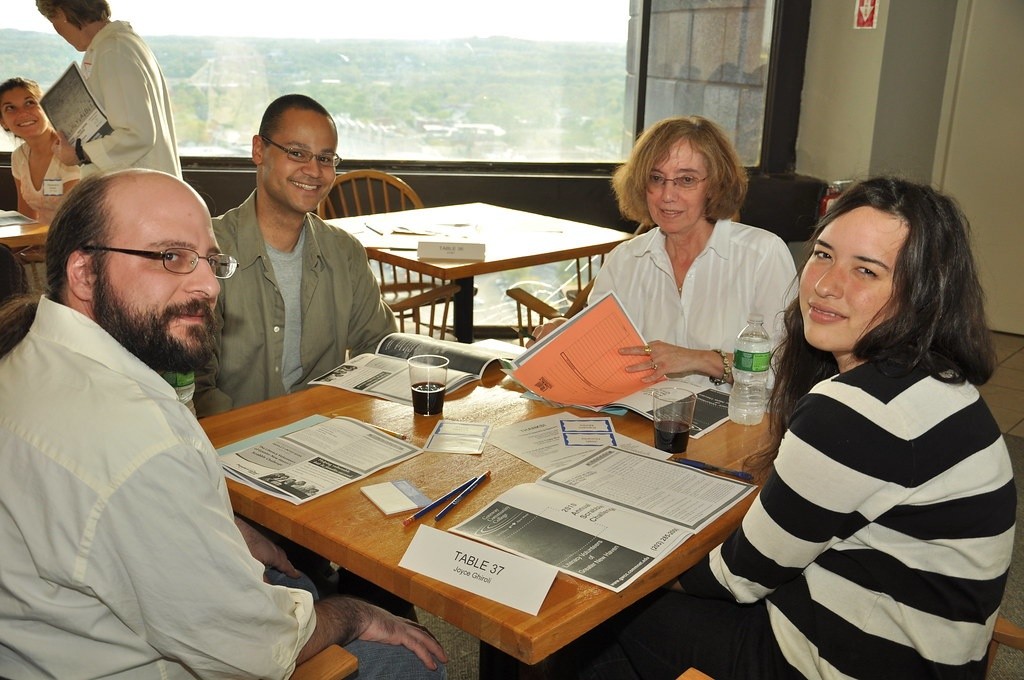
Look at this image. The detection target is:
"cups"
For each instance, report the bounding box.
[651,387,697,455]
[407,353,450,417]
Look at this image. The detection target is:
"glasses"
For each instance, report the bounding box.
[261,136,343,167]
[81,246,240,279]
[648,172,710,189]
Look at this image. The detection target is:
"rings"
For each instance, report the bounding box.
[650,359,658,369]
[645,345,652,355]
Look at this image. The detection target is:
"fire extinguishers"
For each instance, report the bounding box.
[820,179,857,218]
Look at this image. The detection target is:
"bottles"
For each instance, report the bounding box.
[727,313,771,426]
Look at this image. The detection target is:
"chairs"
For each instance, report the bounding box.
[317,170,652,348]
[741,170,826,244]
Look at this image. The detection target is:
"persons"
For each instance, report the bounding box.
[196,94,399,419]
[29,0,183,184]
[668,172,1017,680]
[0,167,447,679]
[526,115,800,396]
[0,78,93,294]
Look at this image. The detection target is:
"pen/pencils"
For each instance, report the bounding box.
[434,470,491,522]
[329,413,407,441]
[403,477,477,526]
[364,222,383,236]
[538,230,563,234]
[389,248,417,251]
[509,324,538,342]
[669,456,754,482]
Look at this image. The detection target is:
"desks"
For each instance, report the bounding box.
[0,222,50,247]
[200,338,788,680]
[323,201,636,345]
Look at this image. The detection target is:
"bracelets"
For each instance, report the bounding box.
[709,349,731,386]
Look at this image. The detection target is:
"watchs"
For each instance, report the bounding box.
[75,138,91,166]
